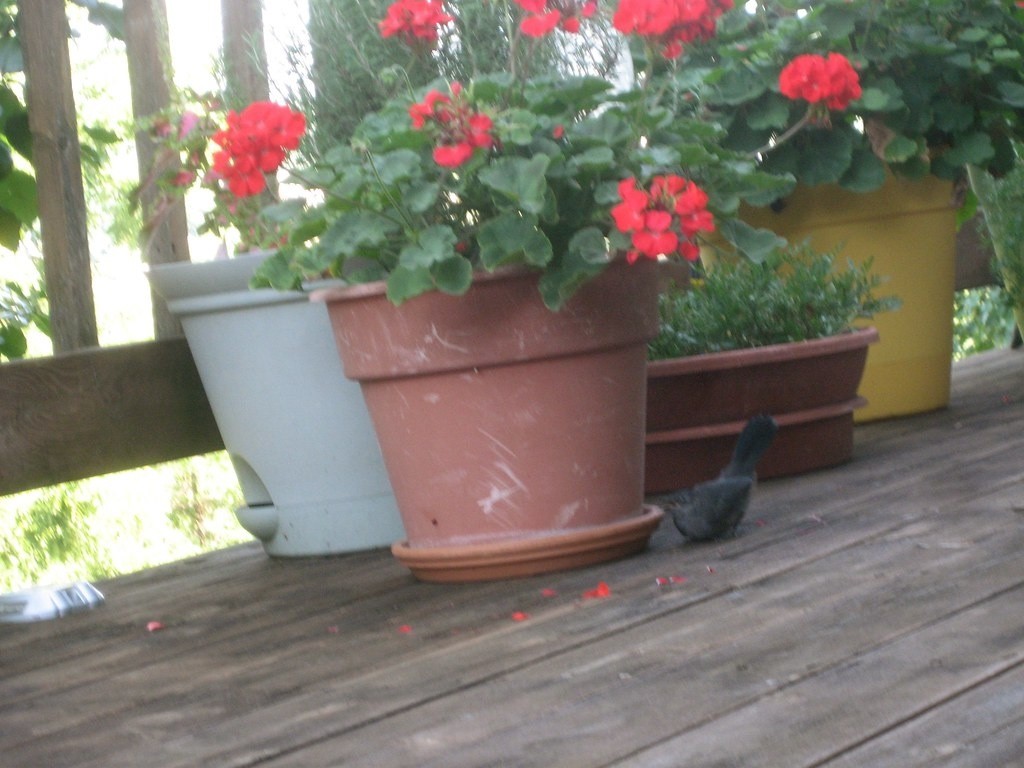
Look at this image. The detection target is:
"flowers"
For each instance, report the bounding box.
[108,47,302,250]
[612,1,1024,216]
[204,0,863,315]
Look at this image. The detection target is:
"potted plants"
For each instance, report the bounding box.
[643,228,903,495]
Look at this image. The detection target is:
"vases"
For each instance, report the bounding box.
[307,245,665,586]
[142,246,411,559]
[694,167,960,429]
